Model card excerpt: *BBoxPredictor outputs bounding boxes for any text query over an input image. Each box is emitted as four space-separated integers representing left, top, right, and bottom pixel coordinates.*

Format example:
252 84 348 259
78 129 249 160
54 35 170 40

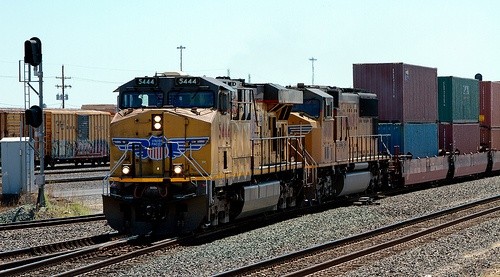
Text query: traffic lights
25 105 43 129
24 37 44 69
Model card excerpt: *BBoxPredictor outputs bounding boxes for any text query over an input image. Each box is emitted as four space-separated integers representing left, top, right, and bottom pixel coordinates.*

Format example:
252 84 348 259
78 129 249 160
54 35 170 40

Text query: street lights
309 58 318 85
177 46 188 72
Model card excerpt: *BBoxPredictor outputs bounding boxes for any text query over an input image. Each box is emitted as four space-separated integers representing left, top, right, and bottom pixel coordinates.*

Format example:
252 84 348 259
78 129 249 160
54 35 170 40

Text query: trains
100 62 500 242
0 106 112 167
